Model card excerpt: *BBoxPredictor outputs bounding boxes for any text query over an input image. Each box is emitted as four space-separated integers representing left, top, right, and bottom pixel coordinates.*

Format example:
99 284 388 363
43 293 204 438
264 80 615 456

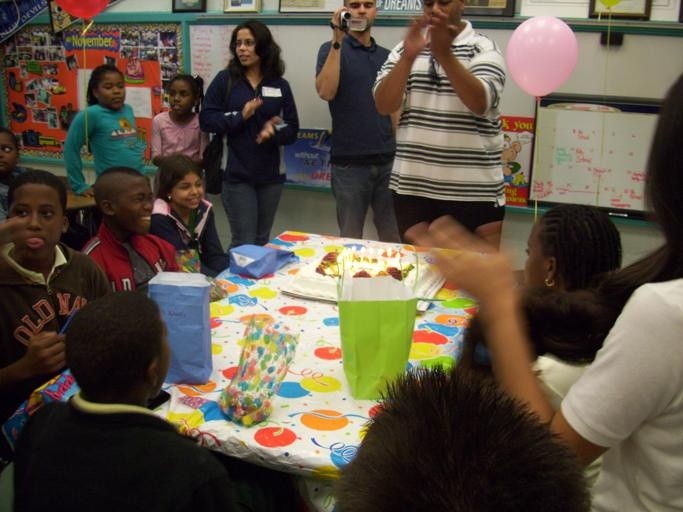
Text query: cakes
286 244 437 308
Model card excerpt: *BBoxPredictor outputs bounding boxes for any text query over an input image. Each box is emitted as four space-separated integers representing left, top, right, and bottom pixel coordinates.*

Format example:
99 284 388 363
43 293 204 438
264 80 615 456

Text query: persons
62 63 159 197
333 362 592 512
199 19 300 251
11 289 304 512
314 0 403 244
2 171 113 418
1 214 44 244
403 72 682 512
372 1 506 252
82 167 228 300
150 155 230 277
520 204 622 413
149 73 211 167
0 126 32 219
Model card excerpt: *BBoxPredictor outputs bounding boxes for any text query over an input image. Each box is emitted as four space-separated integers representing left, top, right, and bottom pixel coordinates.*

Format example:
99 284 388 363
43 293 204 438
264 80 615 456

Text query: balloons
505 15 579 99
54 0 110 20
598 0 621 10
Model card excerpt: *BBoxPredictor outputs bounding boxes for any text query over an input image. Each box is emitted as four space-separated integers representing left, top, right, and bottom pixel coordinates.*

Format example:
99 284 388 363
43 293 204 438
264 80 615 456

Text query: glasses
428 55 440 81
236 41 255 47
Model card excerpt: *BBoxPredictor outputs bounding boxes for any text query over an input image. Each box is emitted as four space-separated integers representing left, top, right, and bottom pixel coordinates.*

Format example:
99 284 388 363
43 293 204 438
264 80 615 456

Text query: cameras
340 10 368 32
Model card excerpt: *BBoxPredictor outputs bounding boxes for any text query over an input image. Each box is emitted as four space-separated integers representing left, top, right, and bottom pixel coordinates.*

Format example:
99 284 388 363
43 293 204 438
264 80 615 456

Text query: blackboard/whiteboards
181 15 683 230
528 93 665 219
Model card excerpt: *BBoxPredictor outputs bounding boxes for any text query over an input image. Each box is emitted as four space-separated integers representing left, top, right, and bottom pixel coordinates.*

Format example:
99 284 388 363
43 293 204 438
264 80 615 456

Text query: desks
65 192 99 211
2 231 479 512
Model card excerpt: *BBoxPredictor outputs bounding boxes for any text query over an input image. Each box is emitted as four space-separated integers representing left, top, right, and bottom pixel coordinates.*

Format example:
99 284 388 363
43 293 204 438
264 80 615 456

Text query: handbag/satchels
202 135 222 195
336 243 420 400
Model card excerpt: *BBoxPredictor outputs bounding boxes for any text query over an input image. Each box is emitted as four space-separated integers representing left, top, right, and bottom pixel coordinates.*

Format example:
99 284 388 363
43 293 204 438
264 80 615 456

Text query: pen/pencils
60 309 77 334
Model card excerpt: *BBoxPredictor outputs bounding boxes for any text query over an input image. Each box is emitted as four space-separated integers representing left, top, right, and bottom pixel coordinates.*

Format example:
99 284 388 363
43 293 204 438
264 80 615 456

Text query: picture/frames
589 0 652 20
279 0 345 13
172 0 206 12
224 0 261 13
461 0 514 17
